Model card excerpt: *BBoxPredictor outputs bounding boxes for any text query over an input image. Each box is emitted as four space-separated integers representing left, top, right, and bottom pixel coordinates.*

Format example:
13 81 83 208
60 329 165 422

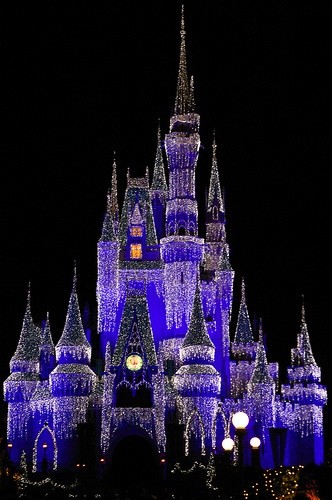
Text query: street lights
219 436 235 500
231 410 249 500
249 436 261 466
